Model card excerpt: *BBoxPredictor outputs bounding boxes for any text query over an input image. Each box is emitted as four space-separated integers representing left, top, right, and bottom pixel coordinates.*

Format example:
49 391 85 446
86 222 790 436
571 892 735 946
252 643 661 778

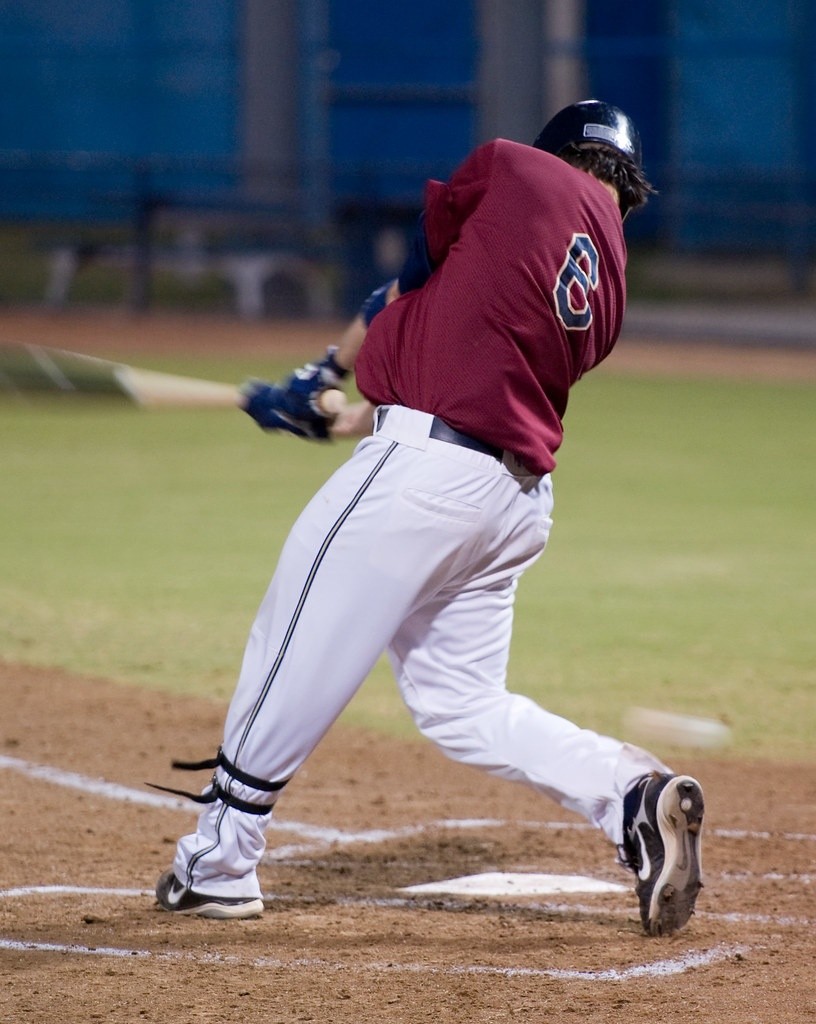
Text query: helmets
532 99 643 165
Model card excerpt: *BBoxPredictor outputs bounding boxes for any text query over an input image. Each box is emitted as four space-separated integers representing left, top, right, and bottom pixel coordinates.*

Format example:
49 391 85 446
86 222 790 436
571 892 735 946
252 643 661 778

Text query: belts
377 407 504 460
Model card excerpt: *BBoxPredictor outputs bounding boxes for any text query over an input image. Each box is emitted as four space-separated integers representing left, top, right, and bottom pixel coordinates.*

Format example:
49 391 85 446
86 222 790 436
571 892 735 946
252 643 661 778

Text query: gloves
285 344 350 394
233 376 337 443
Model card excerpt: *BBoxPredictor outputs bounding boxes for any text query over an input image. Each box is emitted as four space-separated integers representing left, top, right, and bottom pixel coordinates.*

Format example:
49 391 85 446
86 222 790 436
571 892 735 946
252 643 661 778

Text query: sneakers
155 866 265 920
616 770 704 940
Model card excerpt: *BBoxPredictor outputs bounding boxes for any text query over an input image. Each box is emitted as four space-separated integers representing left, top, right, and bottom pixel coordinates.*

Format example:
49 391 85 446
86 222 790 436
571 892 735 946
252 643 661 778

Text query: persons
155 100 704 938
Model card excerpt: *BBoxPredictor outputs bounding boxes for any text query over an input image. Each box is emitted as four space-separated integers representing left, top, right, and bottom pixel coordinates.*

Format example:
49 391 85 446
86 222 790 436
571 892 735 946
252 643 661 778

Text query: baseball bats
1 341 350 416
